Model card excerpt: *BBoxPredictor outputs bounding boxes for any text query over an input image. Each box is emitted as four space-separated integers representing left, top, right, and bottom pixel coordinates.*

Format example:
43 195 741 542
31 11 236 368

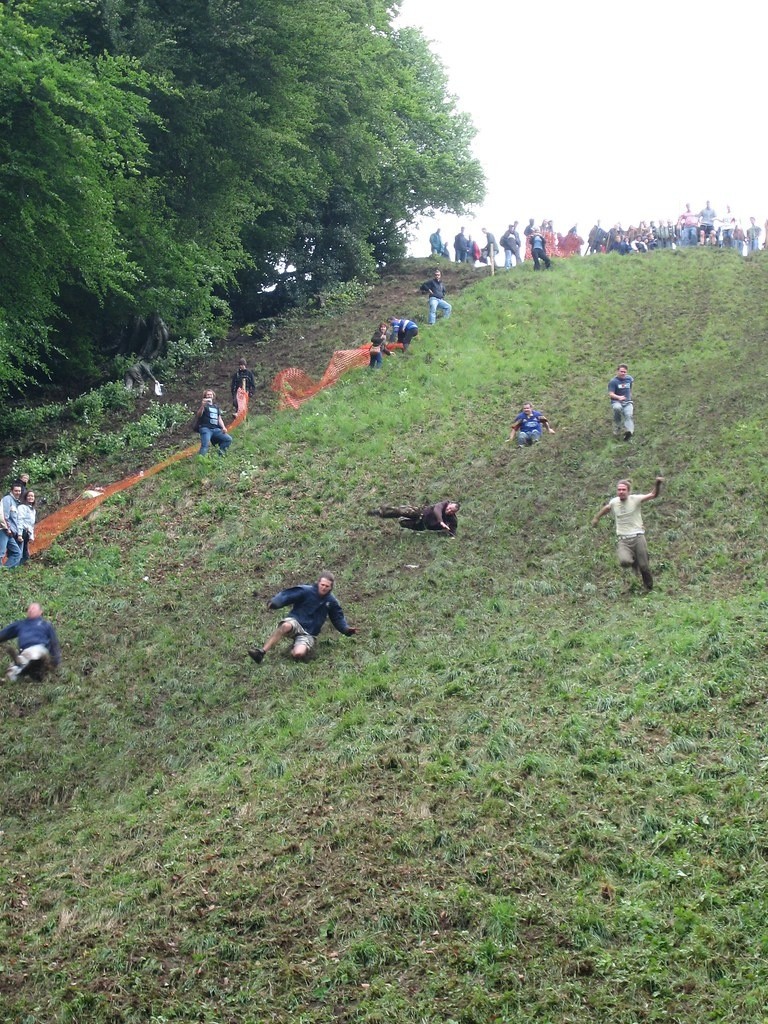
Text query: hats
238 358 247 365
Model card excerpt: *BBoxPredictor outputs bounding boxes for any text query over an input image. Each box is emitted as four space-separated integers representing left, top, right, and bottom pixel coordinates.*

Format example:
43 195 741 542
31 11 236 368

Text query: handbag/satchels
370 346 380 356
155 382 163 396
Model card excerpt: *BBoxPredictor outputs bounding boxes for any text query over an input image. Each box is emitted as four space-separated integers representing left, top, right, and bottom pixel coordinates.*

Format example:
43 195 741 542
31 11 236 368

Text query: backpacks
192 404 218 433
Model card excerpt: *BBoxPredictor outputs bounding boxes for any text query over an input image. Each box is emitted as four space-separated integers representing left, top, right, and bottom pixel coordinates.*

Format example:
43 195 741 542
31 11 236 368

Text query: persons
367 501 460 539
0 601 61 682
505 401 555 448
231 358 255 418
592 477 665 591
422 270 452 325
0 473 36 568
368 316 418 368
248 572 361 665
430 200 761 271
608 364 634 440
197 388 232 456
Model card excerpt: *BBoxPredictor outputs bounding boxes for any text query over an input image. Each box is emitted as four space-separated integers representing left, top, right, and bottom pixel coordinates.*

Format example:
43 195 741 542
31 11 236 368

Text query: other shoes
7 647 22 667
248 649 263 664
139 393 145 399
624 431 631 441
367 508 381 516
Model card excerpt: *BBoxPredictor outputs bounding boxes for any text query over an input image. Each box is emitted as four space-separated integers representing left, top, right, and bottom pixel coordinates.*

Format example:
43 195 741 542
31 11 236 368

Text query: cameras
205 399 212 403
